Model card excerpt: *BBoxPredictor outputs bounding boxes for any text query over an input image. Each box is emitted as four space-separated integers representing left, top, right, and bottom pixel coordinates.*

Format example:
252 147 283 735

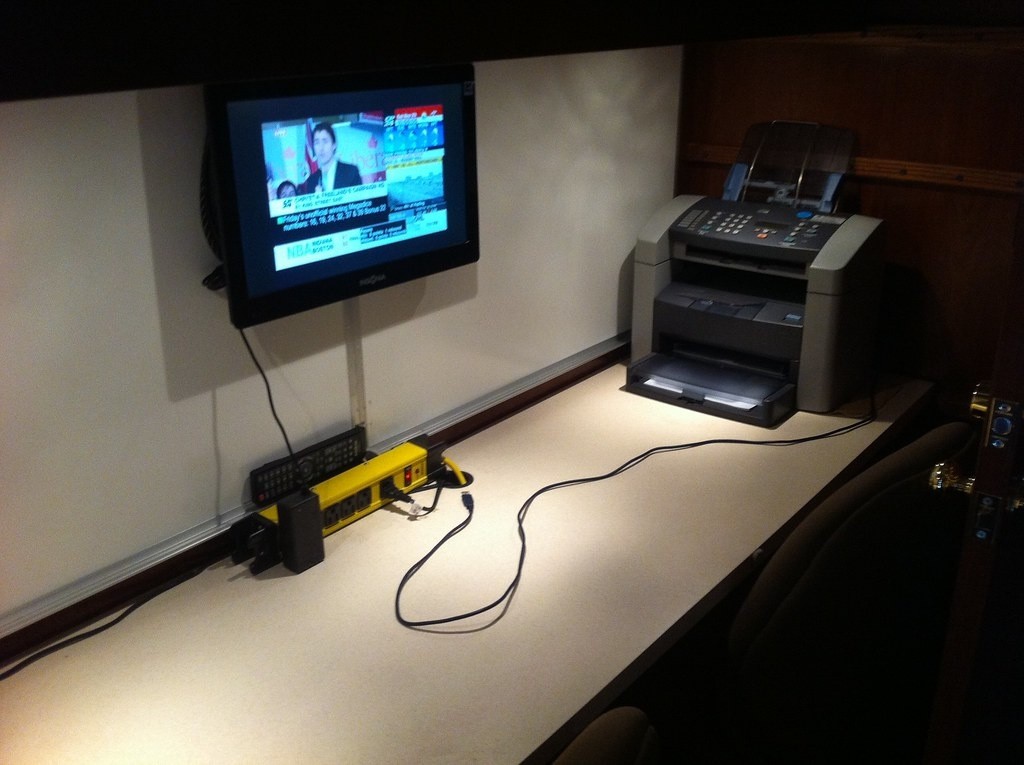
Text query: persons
300 122 361 195
277 182 297 200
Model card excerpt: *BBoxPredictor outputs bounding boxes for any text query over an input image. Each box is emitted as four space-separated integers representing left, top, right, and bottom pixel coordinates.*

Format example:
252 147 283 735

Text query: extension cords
253 434 447 551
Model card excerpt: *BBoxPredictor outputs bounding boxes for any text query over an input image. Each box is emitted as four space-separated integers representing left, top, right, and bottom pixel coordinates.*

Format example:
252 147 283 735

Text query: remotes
248 424 368 509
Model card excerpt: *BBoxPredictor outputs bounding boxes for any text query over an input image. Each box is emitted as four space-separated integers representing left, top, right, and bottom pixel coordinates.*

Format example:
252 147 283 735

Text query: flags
303 118 318 181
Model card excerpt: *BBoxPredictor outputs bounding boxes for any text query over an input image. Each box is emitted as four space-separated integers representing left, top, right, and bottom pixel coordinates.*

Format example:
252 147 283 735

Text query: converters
271 489 328 575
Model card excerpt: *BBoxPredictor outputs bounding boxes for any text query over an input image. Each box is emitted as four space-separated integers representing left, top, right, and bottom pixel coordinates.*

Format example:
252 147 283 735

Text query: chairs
552 706 659 765
726 421 978 663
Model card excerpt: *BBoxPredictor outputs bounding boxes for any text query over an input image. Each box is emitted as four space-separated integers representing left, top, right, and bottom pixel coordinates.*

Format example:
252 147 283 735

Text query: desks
0 352 932 765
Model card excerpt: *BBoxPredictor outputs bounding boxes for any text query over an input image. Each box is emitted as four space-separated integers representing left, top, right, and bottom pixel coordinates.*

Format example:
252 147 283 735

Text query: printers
619 116 888 430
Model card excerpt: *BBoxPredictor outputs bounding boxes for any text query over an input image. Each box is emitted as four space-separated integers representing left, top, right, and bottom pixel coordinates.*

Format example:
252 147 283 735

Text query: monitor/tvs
203 61 482 332
